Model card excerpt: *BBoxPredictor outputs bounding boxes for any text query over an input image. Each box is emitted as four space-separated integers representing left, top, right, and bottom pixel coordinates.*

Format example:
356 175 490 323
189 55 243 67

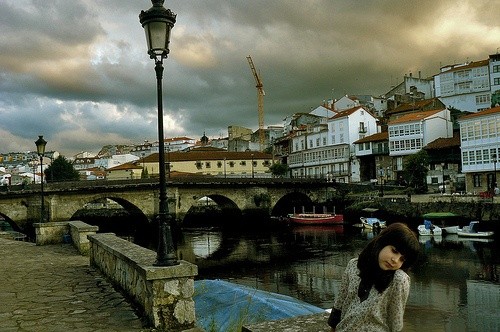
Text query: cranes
244 54 267 153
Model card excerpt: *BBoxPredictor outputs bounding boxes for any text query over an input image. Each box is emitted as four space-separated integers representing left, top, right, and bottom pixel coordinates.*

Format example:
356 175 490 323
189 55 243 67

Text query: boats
417 219 460 236
286 213 344 225
359 216 387 230
456 220 495 238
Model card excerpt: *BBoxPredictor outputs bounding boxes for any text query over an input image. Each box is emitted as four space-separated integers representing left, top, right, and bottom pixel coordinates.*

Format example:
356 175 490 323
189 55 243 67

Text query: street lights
34 135 48 223
138 0 182 267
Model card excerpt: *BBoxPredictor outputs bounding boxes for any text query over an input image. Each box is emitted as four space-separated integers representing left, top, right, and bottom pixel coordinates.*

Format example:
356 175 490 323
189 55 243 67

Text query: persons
327 222 421 332
